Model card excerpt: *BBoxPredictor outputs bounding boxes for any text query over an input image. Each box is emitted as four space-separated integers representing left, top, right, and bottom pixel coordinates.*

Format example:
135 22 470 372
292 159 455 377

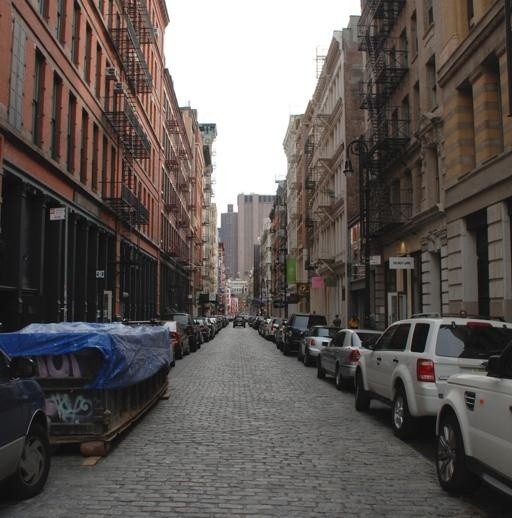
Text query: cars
247 315 288 350
128 312 232 359
300 325 340 368
233 316 246 329
318 329 386 390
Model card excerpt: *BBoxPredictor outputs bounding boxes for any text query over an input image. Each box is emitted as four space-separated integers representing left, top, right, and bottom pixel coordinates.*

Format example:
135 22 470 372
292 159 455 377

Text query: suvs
281 314 329 356
352 312 509 438
0 349 57 506
434 344 512 496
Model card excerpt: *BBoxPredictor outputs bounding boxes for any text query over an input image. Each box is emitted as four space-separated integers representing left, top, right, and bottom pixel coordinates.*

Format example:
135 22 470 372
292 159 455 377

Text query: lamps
342 140 368 178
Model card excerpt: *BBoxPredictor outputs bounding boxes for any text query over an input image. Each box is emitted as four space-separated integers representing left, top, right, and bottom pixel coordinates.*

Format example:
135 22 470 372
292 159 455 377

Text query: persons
333 314 342 328
348 315 359 329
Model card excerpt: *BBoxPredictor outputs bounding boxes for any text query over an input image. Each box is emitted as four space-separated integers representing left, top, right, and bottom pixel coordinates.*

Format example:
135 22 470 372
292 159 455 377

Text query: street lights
279 244 289 316
342 138 374 328
